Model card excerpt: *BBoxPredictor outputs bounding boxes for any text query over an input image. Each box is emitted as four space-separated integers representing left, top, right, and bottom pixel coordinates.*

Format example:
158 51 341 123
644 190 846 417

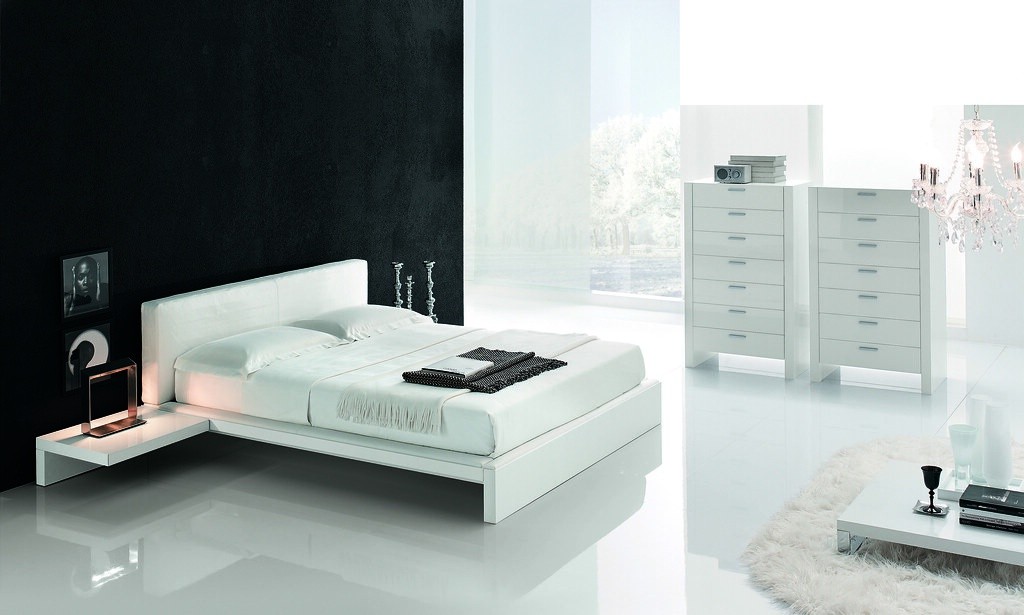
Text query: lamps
909 104 1024 258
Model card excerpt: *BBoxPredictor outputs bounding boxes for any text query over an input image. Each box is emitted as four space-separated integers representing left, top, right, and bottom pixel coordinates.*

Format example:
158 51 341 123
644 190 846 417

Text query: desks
837 456 1024 567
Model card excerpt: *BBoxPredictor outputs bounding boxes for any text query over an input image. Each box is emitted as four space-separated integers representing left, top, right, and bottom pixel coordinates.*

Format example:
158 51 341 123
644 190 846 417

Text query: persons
70 257 109 307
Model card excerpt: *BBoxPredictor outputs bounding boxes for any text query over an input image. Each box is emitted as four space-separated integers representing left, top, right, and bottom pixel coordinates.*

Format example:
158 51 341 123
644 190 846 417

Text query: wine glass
921 466 943 513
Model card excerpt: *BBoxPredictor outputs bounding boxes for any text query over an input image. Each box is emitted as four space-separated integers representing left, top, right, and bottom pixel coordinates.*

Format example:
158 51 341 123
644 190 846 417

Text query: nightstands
36 404 209 487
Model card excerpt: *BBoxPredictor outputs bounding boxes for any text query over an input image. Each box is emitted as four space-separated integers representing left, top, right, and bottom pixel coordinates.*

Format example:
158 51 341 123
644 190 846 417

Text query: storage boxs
938 469 1024 502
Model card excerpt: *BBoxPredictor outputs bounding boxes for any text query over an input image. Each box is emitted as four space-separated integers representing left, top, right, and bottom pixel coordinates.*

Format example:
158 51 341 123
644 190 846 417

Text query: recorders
714 165 751 183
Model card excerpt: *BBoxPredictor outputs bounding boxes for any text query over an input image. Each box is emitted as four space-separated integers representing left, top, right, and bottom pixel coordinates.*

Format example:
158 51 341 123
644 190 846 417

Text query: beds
138 259 663 524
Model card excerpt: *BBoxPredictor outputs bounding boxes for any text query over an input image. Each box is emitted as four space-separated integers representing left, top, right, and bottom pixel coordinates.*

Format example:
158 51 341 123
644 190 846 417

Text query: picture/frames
63 323 113 393
58 246 113 323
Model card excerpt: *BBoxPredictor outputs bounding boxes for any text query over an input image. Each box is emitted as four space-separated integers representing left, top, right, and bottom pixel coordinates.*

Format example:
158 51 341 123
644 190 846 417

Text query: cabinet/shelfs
809 185 948 396
684 176 810 384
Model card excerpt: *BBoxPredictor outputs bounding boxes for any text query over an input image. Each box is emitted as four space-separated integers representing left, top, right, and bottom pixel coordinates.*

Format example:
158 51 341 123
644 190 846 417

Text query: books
727 154 787 183
957 484 1024 534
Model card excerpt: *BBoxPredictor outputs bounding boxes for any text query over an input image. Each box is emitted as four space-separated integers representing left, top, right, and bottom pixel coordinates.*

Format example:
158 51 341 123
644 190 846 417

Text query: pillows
291 302 433 344
172 323 349 380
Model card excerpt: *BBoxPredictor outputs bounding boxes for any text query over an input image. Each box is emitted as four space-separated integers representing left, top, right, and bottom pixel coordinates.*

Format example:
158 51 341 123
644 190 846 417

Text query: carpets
744 433 1024 615
